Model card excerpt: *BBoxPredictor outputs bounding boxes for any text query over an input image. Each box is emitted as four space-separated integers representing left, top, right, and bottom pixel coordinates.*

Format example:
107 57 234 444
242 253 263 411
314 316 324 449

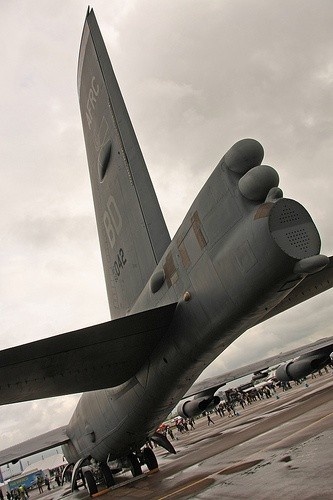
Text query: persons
207 412 215 426
256 386 271 400
294 377 306 386
162 430 166 436
45 474 51 490
6 484 30 500
175 418 195 433
146 441 157 449
53 467 71 486
318 370 322 376
167 427 175 440
324 367 328 373
215 404 236 417
271 382 278 399
37 478 43 494
311 373 316 379
328 364 333 369
239 394 257 409
281 382 292 392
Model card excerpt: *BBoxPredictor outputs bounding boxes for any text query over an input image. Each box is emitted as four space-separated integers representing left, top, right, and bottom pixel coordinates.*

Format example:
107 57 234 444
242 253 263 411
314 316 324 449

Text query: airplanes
0 3 333 496
